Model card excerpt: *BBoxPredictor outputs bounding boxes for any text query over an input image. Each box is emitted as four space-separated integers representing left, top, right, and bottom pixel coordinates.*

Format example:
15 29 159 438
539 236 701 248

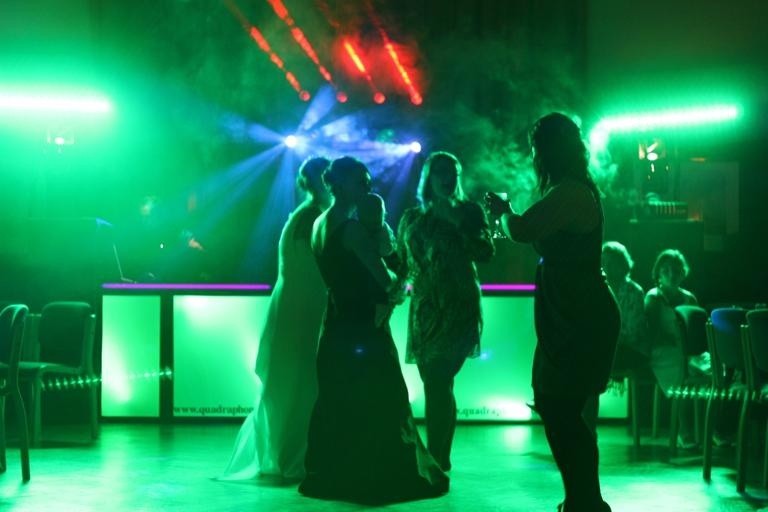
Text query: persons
235 158 335 481
355 193 403 329
638 247 699 310
480 111 624 512
396 149 496 472
301 154 452 505
523 237 645 416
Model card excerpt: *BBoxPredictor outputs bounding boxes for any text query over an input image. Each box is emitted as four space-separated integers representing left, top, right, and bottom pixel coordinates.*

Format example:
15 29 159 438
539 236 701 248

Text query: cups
488 191 508 240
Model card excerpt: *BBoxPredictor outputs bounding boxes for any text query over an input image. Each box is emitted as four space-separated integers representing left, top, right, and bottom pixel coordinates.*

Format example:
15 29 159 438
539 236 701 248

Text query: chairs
622 304 767 494
0 301 98 483
